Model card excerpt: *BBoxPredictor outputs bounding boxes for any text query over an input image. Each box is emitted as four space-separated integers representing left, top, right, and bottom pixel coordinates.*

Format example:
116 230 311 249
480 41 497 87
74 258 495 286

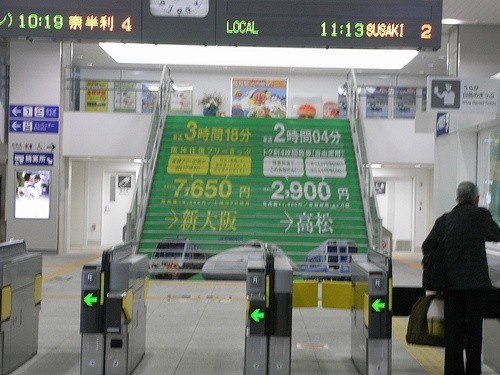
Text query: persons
23 173 39 199
33 175 43 197
420 181 499 375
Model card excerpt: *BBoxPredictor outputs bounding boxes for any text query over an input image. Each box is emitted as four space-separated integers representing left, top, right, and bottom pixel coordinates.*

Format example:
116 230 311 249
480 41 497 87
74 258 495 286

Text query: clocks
149 0 209 18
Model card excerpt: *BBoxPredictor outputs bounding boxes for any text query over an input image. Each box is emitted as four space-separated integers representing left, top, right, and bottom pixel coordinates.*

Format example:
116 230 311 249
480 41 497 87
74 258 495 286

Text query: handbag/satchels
405 252 447 347
